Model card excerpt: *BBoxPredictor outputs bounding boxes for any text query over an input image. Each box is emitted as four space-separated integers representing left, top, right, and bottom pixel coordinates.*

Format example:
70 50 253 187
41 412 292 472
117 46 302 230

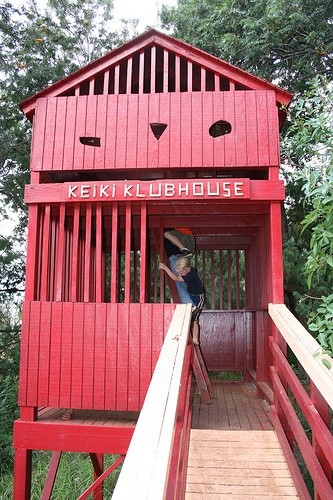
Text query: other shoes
180 249 193 257
192 307 202 320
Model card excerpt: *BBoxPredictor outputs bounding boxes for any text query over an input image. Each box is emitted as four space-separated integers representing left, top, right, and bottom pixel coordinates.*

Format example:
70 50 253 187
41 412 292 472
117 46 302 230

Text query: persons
159 257 205 345
163 227 200 316
